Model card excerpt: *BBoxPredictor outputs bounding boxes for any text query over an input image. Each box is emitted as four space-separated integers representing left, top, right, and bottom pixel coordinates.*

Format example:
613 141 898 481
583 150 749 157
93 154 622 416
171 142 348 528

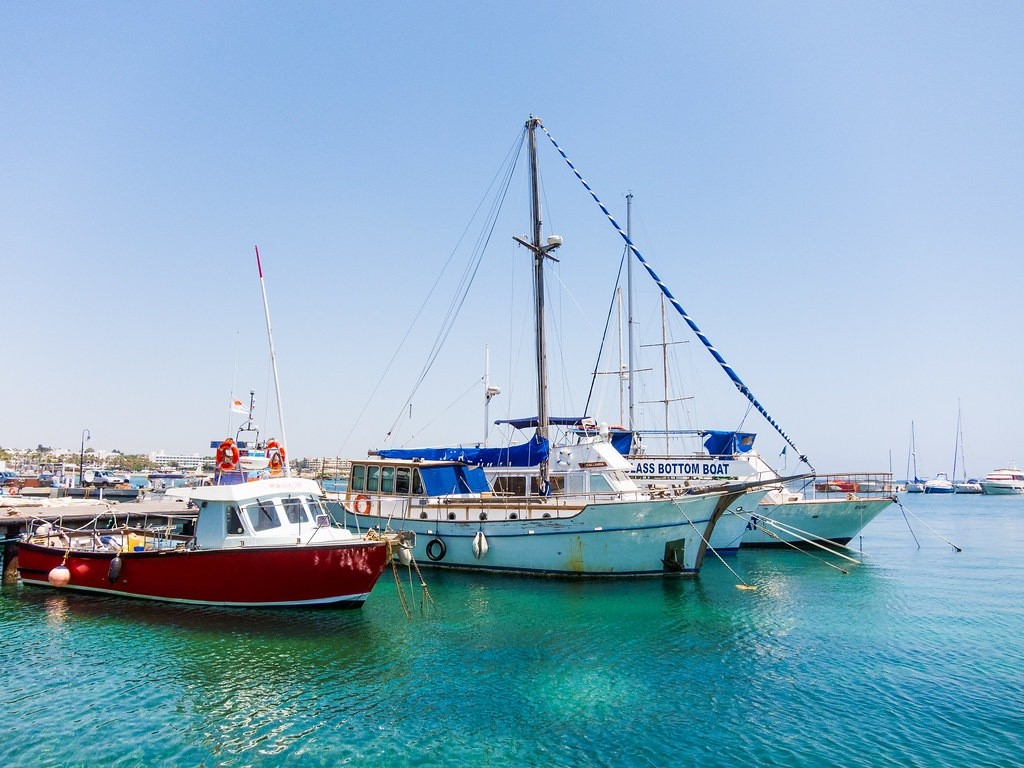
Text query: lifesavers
557 449 572 466
216 441 239 471
265 441 286 468
9 487 16 495
353 495 370 514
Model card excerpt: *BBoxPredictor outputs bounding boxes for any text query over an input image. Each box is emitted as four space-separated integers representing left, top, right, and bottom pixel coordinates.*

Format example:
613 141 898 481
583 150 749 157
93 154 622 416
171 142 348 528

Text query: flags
230 391 251 414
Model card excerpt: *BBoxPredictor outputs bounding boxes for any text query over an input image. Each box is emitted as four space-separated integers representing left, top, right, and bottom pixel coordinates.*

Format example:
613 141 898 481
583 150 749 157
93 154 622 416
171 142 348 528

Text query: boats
979 459 1024 495
950 398 984 493
562 287 896 551
811 419 925 493
1 114 816 607
924 473 956 493
